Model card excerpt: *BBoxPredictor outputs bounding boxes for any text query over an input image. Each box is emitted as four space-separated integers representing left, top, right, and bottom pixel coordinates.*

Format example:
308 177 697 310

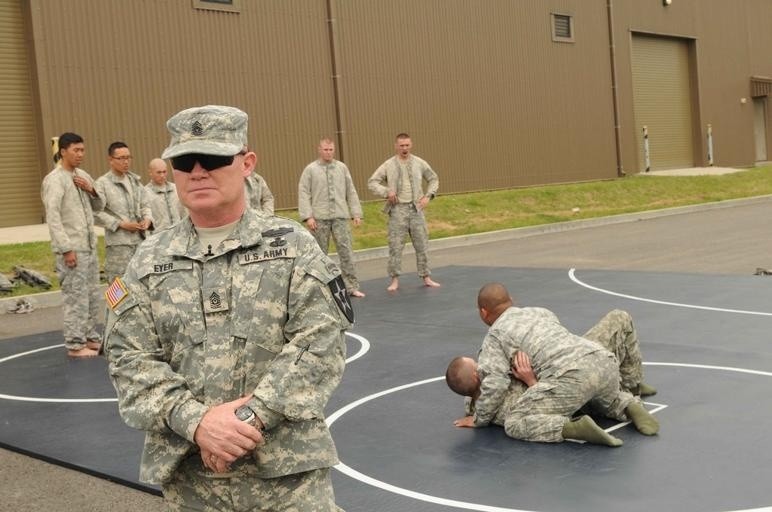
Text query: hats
161 103 251 160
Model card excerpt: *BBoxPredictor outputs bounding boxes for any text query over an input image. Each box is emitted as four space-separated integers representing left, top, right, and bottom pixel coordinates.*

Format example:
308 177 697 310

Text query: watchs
235 405 263 435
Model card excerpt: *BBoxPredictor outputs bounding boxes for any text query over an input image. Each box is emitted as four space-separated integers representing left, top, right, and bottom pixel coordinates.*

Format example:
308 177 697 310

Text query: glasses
170 154 235 173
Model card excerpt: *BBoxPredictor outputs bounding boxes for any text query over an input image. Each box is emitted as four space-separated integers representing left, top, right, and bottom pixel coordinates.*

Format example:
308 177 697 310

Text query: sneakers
5 294 37 314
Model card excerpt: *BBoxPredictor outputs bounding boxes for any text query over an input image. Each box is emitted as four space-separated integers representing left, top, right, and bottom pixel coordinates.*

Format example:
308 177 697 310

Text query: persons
40 132 188 357
446 308 657 426
367 133 441 292
104 105 356 512
297 137 364 298
246 173 275 217
453 283 659 448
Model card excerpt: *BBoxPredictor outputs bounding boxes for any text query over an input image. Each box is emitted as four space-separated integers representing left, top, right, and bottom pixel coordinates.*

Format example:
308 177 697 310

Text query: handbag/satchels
0 271 21 290
12 262 55 292
